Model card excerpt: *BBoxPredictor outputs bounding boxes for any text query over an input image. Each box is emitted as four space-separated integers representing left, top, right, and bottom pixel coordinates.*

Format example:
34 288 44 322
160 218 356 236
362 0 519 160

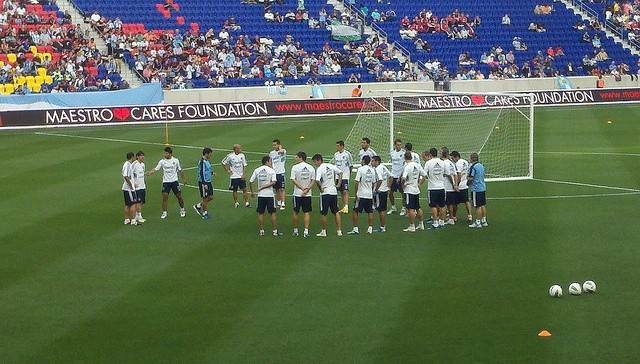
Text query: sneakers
316 229 327 237
192 204 203 215
468 215 473 221
337 230 343 237
347 227 359 235
416 224 424 230
426 216 433 221
124 218 130 224
161 211 168 219
469 222 482 228
234 202 240 208
426 220 434 224
272 229 283 236
482 221 488 226
427 224 441 230
373 226 386 233
202 212 211 220
180 210 186 217
403 226 415 232
386 207 397 215
399 207 406 216
277 201 281 208
439 224 444 228
280 202 286 211
131 219 142 226
138 217 146 223
259 230 265 236
246 202 253 208
366 226 373 234
339 207 345 213
304 229 309 237
293 228 299 236
343 207 348 214
444 219 455 225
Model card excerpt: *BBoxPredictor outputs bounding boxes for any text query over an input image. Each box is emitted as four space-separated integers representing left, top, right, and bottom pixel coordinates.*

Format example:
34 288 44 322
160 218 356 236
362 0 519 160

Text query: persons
269 138 287 210
191 147 217 220
346 154 378 234
289 151 316 237
1 0 126 94
466 152 489 228
597 74 605 88
404 141 420 163
349 0 589 81
311 153 344 236
221 143 250 208
249 155 284 236
145 146 188 218
399 151 428 232
334 139 354 213
120 151 138 225
351 84 362 96
358 136 377 162
590 0 640 74
437 146 459 225
369 155 394 232
419 147 451 228
386 138 412 215
126 0 349 87
131 149 147 223
449 150 473 222
421 150 431 161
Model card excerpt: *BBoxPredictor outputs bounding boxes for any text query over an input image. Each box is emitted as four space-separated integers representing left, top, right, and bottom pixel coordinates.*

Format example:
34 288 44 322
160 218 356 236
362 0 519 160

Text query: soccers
583 280 597 293
568 282 582 295
550 285 563 296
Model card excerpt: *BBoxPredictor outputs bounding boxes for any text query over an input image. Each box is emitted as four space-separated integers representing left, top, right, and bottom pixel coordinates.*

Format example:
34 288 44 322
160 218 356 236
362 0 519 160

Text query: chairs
0 0 130 94
574 0 612 26
